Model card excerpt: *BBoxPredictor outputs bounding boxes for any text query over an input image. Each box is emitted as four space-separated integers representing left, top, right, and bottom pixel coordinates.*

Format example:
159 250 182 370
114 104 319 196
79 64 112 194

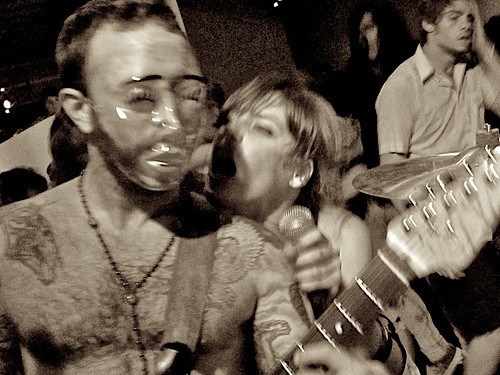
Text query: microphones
280 203 330 328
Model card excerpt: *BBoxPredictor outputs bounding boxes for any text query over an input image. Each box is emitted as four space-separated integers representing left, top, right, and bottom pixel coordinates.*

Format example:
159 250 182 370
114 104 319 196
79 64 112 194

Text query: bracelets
483 43 496 74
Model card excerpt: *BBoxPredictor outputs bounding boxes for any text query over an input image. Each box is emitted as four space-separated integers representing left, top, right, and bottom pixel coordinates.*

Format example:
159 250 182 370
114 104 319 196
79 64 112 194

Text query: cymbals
352 146 488 202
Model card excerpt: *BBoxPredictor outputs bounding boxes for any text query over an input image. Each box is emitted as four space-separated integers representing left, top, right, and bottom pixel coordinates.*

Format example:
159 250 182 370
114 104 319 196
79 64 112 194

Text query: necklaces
79 166 186 375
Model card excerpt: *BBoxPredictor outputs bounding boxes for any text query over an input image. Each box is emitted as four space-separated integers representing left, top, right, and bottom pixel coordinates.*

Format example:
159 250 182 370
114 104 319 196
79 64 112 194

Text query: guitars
264 148 500 375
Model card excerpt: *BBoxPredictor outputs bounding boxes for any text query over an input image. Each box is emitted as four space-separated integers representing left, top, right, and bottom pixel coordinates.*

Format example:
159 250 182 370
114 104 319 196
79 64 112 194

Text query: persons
0 87 90 210
205 69 420 375
374 0 500 338
0 0 388 375
359 8 413 62
